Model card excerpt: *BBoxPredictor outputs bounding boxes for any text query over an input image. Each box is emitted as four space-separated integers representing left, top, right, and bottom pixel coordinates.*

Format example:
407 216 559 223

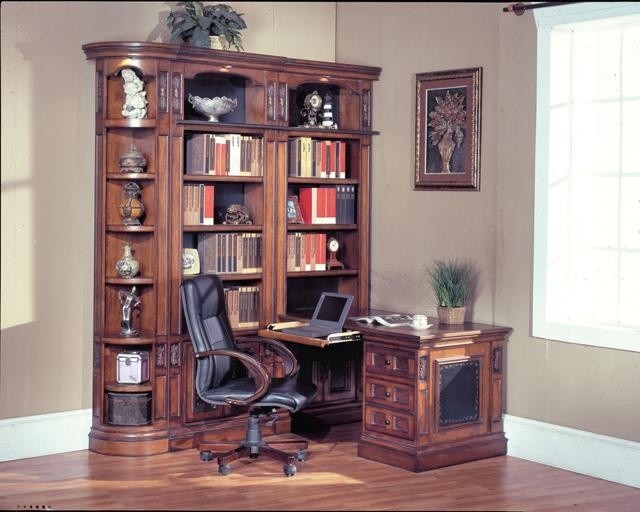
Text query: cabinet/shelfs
82 41 170 458
171 46 277 453
276 58 382 435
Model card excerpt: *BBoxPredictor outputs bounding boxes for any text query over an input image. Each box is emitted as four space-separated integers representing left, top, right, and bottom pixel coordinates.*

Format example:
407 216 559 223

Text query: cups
413 315 428 325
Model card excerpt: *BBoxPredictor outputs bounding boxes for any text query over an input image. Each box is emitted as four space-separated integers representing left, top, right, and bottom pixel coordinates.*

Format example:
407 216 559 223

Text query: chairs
178 275 307 477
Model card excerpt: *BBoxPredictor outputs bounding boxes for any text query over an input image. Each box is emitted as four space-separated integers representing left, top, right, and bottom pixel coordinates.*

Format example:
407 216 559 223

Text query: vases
438 133 456 173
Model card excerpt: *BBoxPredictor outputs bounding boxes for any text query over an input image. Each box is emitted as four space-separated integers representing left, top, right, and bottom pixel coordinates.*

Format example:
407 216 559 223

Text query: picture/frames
411 66 483 191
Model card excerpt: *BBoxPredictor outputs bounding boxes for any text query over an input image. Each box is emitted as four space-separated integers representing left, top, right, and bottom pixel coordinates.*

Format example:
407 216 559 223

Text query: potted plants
164 1 248 52
423 255 478 324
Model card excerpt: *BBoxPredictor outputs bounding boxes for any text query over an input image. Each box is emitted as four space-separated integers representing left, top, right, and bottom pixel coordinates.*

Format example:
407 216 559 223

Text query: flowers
427 90 466 148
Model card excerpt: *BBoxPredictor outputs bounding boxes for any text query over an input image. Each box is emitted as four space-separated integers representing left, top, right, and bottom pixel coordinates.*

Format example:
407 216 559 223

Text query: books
350 311 411 327
187 132 263 330
288 136 355 272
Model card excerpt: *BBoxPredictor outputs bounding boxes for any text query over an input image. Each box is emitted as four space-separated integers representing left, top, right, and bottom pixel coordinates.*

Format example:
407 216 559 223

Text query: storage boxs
116 351 149 385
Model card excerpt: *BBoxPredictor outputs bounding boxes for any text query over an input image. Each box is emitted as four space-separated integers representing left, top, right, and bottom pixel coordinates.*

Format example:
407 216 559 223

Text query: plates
408 324 433 330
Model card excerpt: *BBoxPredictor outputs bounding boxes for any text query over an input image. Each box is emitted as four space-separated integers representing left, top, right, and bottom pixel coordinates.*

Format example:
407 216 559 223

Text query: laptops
282 291 354 338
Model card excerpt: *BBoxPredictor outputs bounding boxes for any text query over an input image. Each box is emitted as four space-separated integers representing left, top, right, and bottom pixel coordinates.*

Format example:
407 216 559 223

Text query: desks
277 307 513 473
